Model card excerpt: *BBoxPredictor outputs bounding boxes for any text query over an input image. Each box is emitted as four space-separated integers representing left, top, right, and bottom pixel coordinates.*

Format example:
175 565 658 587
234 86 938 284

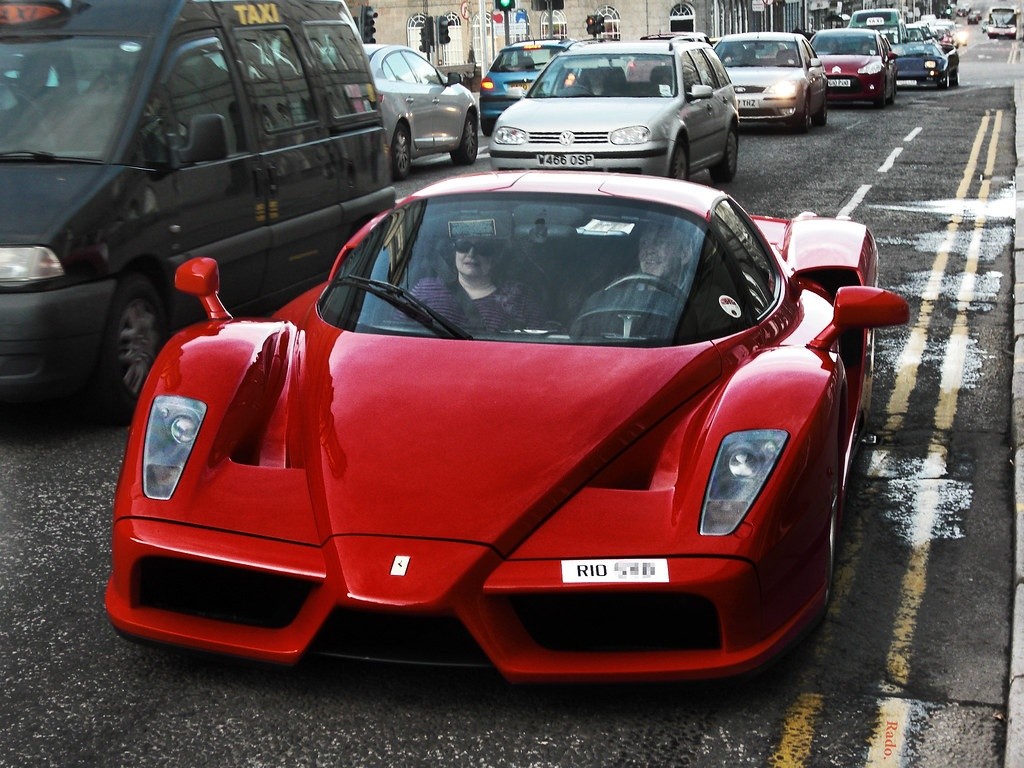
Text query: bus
986 7 1020 41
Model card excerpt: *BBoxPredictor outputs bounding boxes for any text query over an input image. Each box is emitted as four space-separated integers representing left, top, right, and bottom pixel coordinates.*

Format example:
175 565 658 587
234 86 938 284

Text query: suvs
479 36 582 139
487 34 740 186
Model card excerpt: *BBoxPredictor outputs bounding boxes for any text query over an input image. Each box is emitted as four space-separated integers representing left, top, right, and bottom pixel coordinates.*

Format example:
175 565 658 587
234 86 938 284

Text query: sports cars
102 166 914 692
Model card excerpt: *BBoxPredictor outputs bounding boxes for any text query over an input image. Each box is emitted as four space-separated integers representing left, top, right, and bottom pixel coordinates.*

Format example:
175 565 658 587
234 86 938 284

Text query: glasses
452 239 498 253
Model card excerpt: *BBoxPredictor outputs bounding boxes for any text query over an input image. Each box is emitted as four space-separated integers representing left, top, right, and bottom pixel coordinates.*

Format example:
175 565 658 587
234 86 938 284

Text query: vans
0 1 401 424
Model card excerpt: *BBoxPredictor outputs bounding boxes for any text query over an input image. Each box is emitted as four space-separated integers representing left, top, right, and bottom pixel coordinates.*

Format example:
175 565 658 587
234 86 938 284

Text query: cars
809 27 900 107
706 32 831 135
966 14 978 26
973 10 982 19
982 21 989 33
626 32 715 84
353 45 482 178
844 4 972 90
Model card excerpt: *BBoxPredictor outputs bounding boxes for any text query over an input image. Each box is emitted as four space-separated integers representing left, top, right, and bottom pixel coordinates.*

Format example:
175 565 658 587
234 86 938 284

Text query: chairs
597 67 626 95
519 56 534 70
646 66 672 94
433 210 546 322
740 47 756 64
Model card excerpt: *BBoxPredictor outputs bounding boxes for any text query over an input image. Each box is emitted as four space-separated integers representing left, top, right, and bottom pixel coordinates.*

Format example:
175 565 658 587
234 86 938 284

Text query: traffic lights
417 27 424 53
423 16 434 47
594 16 606 35
495 0 515 11
586 16 596 37
438 16 450 45
358 4 377 46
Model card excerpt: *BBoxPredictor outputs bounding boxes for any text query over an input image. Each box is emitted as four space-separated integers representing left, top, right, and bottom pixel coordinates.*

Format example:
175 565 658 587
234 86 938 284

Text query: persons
579 62 619 96
604 219 729 339
397 216 548 334
